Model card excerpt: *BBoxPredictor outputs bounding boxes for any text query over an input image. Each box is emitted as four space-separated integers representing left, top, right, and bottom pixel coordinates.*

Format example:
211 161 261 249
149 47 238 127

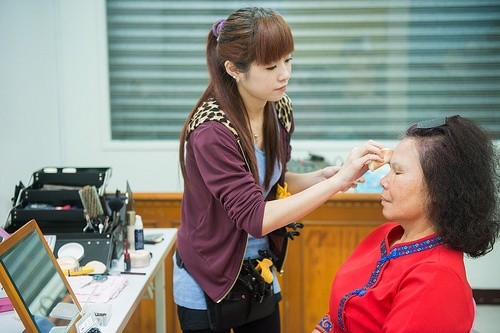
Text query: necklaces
251 127 263 142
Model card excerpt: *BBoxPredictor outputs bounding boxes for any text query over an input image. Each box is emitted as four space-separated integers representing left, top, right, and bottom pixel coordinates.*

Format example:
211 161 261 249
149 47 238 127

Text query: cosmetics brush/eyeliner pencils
83 200 123 233
120 272 146 275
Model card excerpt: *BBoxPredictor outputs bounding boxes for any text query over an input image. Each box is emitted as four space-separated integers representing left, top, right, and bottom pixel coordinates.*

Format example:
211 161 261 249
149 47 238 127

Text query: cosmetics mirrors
0 219 84 333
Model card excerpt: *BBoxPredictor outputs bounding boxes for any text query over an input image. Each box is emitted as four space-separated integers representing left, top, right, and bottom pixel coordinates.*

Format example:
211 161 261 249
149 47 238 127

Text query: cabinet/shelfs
123 193 394 333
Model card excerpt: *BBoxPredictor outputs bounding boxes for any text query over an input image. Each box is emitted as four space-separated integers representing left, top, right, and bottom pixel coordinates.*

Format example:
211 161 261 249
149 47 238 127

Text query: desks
0 228 179 333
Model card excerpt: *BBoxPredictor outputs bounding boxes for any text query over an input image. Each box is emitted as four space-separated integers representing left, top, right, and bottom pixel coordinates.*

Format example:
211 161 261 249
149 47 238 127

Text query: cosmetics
367 147 395 172
124 211 152 272
55 256 95 277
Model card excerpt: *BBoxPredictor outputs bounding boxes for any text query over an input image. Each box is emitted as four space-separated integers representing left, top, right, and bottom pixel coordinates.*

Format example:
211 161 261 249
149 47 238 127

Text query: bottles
127 211 137 250
133 215 144 250
123 250 131 273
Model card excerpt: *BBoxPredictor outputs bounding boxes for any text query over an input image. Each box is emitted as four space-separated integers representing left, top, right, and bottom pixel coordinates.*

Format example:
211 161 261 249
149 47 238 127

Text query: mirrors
0 219 82 333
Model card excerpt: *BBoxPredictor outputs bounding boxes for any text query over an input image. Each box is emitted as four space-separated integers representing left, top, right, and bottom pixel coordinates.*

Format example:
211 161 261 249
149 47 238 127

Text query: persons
309 115 500 333
171 7 388 333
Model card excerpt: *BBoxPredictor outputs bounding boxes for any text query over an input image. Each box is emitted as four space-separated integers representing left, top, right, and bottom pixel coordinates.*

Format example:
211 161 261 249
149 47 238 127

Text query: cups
129 250 151 268
